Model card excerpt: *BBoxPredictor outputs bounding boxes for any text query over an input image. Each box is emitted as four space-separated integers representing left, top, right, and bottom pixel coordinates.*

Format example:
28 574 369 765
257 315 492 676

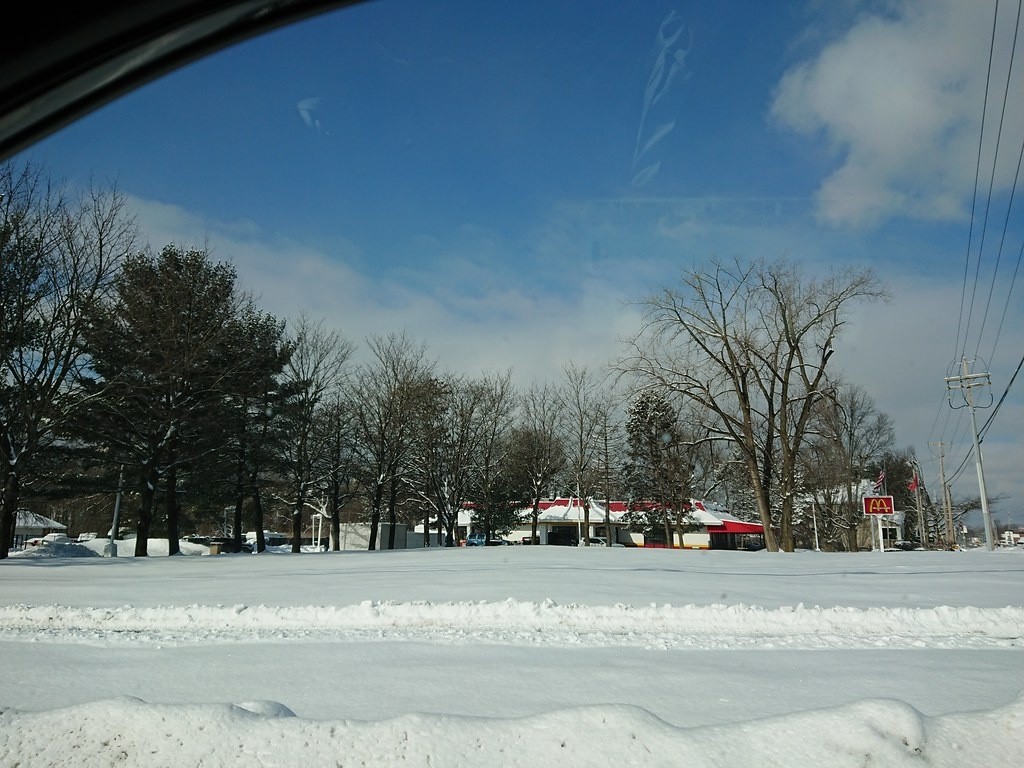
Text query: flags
874 469 885 490
908 473 918 491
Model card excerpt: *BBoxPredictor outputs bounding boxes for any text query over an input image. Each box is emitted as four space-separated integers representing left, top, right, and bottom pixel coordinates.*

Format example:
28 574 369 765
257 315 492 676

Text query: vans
466 532 508 546
108 526 133 540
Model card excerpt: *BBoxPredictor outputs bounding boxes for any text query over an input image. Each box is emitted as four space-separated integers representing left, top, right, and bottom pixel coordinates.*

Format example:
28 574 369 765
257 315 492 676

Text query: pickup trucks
522 532 565 544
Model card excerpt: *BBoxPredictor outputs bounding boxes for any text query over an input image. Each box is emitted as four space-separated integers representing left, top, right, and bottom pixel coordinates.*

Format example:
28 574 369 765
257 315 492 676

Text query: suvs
577 537 625 547
